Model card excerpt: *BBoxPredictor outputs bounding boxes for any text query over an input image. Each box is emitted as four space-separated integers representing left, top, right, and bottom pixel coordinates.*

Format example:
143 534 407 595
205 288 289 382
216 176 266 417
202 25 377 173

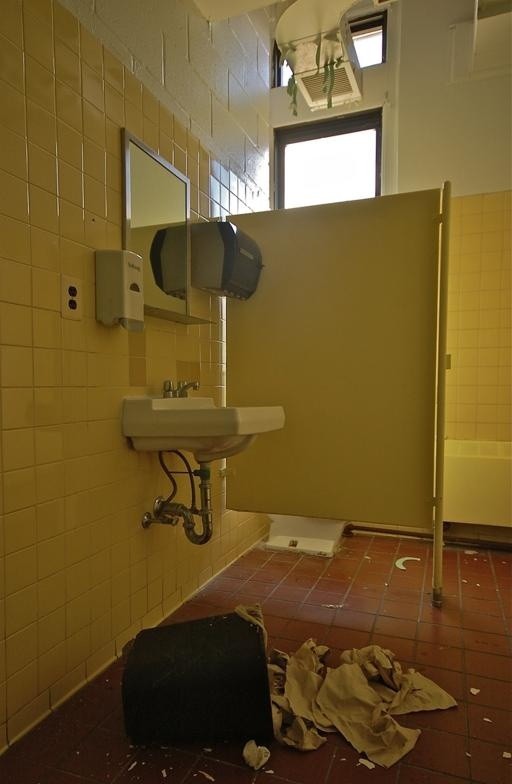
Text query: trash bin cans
122 600 277 751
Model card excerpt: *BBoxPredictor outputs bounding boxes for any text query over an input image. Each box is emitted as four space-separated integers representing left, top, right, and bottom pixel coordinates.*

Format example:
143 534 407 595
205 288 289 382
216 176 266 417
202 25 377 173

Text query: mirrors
119 127 189 326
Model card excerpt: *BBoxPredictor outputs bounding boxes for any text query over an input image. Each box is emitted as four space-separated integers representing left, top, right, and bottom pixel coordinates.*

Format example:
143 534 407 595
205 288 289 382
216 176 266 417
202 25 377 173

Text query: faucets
162 377 206 401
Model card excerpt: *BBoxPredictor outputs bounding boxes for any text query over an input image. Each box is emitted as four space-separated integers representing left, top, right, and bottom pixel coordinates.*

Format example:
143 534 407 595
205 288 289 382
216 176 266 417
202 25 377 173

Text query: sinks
121 395 286 464
